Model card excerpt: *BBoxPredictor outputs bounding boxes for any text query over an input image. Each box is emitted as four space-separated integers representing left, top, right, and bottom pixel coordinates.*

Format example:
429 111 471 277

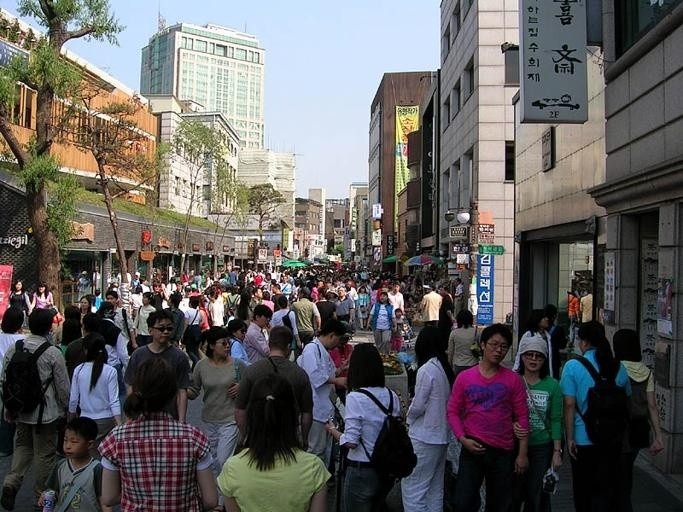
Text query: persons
0 259 664 512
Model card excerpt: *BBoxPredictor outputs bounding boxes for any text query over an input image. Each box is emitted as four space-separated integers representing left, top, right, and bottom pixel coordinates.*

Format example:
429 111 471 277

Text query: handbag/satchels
469 340 482 357
360 304 365 313
282 315 294 330
635 419 652 449
550 433 565 459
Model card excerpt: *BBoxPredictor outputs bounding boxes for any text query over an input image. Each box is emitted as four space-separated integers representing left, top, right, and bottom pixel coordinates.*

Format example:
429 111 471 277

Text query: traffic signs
478 244 506 255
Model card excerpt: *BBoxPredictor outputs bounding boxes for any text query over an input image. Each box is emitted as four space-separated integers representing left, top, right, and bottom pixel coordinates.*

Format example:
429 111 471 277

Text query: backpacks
572 354 630 445
3 339 54 415
354 387 417 477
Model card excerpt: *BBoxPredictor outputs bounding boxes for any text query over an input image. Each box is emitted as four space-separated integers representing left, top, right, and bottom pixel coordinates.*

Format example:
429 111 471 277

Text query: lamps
444 204 477 224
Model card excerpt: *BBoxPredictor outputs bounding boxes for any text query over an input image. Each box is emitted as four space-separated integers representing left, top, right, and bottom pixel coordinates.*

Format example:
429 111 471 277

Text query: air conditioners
372 203 382 220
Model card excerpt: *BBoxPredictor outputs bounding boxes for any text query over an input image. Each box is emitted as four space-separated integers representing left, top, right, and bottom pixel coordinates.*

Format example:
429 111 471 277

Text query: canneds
43 489 55 512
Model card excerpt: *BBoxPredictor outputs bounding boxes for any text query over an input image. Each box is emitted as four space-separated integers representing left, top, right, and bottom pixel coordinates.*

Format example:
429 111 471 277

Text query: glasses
150 325 174 332
238 328 247 334
486 341 509 352
215 340 233 346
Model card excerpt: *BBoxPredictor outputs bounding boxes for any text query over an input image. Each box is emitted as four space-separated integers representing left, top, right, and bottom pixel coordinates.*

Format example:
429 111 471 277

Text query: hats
519 336 548 360
